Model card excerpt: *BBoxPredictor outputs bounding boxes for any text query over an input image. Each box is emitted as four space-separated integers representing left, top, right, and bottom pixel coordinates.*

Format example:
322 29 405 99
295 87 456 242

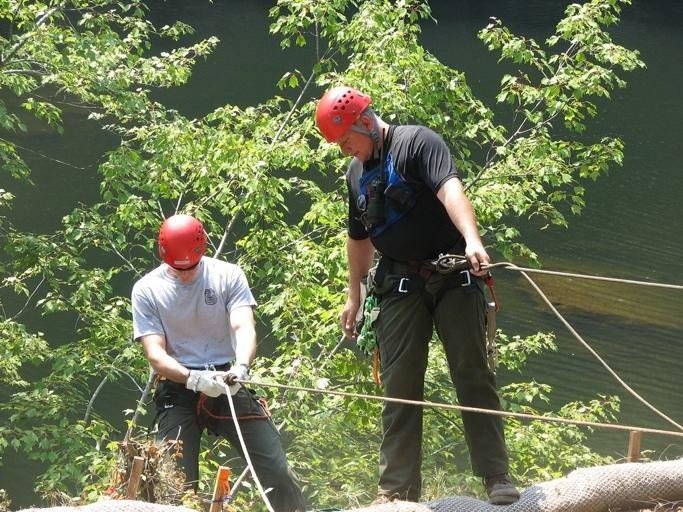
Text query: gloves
186 364 249 399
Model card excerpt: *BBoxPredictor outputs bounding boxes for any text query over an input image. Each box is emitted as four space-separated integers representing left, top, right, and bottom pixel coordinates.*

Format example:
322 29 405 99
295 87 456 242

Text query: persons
129 213 308 512
316 85 522 506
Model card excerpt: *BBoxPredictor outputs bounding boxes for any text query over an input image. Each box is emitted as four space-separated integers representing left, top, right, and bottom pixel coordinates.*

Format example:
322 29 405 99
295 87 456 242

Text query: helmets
158 214 208 270
316 86 372 144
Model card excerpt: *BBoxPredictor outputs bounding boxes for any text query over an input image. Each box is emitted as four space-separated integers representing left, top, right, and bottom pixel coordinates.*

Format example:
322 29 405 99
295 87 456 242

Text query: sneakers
370 495 419 506
483 473 520 503
